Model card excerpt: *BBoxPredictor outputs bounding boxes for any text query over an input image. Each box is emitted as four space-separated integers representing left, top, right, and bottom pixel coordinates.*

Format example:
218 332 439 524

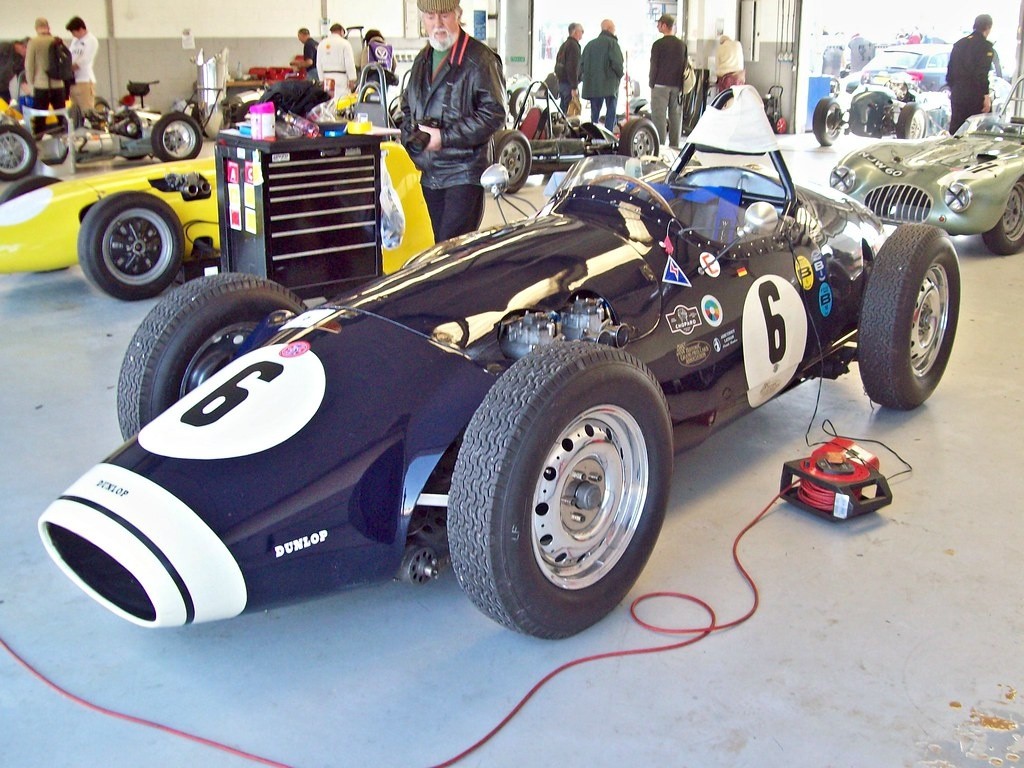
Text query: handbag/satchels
683 66 696 94
567 93 582 115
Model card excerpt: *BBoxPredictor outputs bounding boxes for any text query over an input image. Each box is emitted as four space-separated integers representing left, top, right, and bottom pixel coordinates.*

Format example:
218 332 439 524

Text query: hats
417 0 460 14
656 15 674 26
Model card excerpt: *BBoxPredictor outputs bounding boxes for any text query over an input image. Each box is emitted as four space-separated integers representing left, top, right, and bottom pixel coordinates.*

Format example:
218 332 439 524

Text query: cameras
404 117 445 157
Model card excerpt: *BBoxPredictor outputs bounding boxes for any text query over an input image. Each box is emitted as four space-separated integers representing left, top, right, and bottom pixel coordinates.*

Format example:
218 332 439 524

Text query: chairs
664 84 796 219
354 62 390 141
513 80 553 140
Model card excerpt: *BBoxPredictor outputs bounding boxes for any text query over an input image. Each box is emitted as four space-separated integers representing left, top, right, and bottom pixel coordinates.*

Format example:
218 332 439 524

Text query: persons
946 14 994 135
291 28 319 84
360 31 397 92
649 15 688 147
715 38 745 107
576 20 624 132
0 38 31 105
400 0 507 243
25 18 70 134
315 24 356 97
65 16 99 116
554 23 584 114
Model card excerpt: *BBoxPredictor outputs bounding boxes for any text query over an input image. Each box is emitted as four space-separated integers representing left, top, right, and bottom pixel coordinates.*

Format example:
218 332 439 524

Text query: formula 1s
0 64 962 638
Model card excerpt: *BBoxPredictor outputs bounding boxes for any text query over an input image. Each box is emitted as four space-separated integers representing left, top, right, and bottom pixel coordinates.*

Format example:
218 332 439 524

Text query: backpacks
44 36 73 80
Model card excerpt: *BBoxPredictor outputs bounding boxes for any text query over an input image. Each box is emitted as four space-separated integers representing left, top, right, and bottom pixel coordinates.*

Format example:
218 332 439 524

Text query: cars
812 43 1011 145
829 78 1024 253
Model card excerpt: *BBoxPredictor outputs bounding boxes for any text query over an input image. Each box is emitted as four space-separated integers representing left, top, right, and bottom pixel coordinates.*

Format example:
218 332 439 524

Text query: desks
227 80 275 92
